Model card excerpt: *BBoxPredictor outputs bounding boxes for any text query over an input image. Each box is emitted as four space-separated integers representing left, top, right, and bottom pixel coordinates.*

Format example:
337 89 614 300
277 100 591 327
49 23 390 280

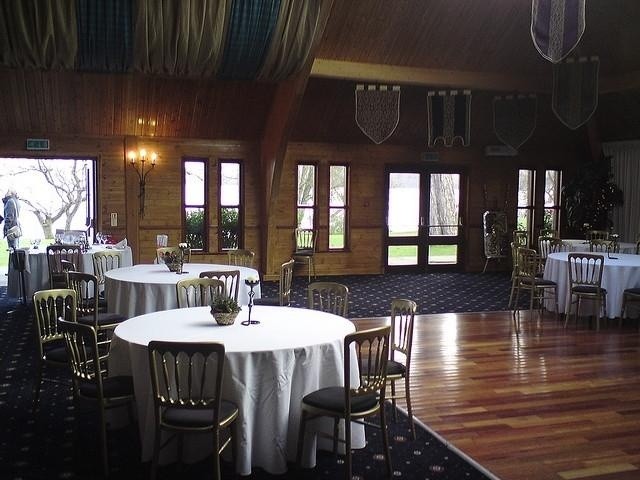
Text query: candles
247 274 258 283
140 149 146 160
128 151 136 163
151 154 156 164
583 222 590 227
178 242 188 249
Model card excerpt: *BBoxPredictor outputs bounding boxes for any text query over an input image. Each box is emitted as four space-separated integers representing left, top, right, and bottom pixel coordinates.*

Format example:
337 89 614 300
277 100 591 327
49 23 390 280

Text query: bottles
58 240 62 246
79 236 84 251
82 237 86 250
85 241 89 249
54 240 58 247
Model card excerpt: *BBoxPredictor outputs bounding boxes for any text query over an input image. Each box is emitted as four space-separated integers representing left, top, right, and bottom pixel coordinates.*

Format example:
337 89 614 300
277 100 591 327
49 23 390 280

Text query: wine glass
36 239 41 248
101 234 107 249
31 239 36 249
96 232 102 248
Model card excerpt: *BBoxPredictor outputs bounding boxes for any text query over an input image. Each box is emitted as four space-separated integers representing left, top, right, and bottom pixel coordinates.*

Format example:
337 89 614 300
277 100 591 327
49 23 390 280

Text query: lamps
241 273 260 326
176 242 189 274
582 222 592 244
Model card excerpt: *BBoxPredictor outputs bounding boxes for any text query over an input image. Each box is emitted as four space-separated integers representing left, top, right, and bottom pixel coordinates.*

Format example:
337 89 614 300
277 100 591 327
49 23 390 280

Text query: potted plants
164 256 184 272
209 294 243 325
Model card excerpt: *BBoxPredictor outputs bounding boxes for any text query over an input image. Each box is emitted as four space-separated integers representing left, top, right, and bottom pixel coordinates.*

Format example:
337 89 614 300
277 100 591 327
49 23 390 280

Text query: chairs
355 298 417 438
147 341 239 480
297 324 393 480
292 228 318 283
59 316 136 480
199 270 241 308
177 278 226 310
227 249 255 268
156 247 191 264
508 229 639 329
46 245 82 289
253 260 292 306
481 223 509 275
308 282 349 317
92 252 129 299
67 270 129 355
32 288 78 403
